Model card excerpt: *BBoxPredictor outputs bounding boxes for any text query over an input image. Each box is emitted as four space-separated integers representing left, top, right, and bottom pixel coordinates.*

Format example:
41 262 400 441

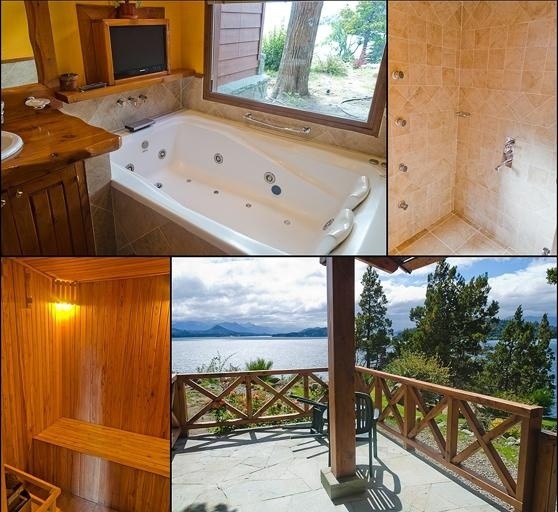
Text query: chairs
283 392 380 468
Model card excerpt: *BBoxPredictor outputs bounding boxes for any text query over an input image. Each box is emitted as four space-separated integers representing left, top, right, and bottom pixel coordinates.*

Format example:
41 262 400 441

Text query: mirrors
1 0 61 97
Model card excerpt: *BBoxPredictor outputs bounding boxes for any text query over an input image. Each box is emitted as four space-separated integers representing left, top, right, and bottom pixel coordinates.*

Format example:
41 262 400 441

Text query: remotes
79 81 105 90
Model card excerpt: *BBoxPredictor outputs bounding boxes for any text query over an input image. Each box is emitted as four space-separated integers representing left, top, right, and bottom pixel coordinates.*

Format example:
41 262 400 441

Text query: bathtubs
108 106 387 255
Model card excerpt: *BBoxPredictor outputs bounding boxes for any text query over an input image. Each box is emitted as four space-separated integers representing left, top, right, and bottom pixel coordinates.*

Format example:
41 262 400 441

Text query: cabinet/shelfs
0 161 95 256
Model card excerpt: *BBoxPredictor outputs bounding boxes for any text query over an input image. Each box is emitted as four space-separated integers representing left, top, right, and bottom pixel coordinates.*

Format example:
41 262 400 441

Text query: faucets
495 159 510 172
0 99 5 124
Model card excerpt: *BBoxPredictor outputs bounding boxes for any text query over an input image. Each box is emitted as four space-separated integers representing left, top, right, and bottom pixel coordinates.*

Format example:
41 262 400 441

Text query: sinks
0 130 25 160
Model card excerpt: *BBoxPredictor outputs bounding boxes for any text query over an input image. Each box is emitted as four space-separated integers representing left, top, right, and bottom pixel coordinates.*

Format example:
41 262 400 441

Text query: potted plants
60 72 80 91
114 0 142 19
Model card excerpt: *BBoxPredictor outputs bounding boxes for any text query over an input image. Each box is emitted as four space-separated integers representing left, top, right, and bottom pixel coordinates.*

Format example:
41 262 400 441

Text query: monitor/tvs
110 25 166 79
